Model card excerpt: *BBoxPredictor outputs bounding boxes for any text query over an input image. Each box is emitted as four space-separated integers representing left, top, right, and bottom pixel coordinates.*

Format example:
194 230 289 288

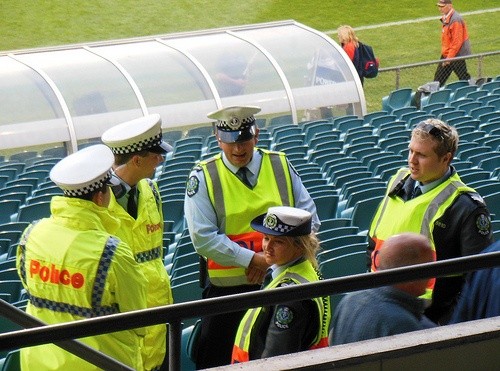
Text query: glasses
417 122 451 152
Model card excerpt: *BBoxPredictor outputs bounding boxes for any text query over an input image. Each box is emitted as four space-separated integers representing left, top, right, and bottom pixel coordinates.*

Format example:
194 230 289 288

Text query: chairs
0 76 500 371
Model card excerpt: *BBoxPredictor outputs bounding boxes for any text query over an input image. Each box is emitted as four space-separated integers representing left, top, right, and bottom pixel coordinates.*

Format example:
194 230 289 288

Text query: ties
412 188 421 199
127 187 137 218
261 271 273 290
239 167 252 188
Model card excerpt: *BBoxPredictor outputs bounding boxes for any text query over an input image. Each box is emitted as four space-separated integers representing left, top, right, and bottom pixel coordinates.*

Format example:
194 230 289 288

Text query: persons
184 105 321 371
366 116 491 327
330 234 437 345
338 25 364 115
232 206 330 362
101 112 174 371
16 144 147 371
214 56 249 97
433 0 473 88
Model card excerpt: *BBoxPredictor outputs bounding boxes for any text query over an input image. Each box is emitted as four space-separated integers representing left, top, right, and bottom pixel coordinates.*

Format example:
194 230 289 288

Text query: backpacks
356 41 379 78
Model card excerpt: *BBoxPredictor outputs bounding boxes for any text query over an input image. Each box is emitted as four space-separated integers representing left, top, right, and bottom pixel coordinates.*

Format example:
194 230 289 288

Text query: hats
206 105 263 142
101 113 174 155
437 0 452 7
249 205 312 236
52 143 121 197
